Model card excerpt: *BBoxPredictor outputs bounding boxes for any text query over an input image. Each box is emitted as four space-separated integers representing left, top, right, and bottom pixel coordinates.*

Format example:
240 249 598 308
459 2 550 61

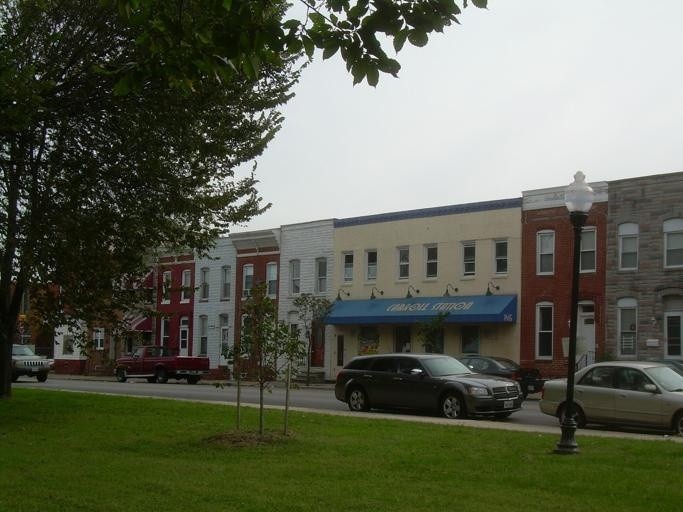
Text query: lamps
486 282 500 296
370 287 383 301
406 285 420 299
443 283 458 297
335 289 350 302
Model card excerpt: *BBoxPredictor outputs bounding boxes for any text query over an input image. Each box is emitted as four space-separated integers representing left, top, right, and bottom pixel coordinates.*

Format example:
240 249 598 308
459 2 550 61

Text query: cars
538 360 683 437
453 353 545 400
644 357 683 371
11 343 54 383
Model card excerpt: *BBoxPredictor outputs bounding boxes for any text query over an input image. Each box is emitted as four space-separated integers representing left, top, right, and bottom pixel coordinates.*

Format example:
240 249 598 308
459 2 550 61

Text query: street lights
553 170 594 455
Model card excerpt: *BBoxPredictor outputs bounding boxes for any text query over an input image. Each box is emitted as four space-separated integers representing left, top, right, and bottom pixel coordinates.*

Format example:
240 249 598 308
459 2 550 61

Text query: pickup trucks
112 345 209 384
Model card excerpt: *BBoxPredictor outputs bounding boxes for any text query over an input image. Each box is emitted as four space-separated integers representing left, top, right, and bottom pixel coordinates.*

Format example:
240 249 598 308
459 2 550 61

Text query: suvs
335 353 524 419
